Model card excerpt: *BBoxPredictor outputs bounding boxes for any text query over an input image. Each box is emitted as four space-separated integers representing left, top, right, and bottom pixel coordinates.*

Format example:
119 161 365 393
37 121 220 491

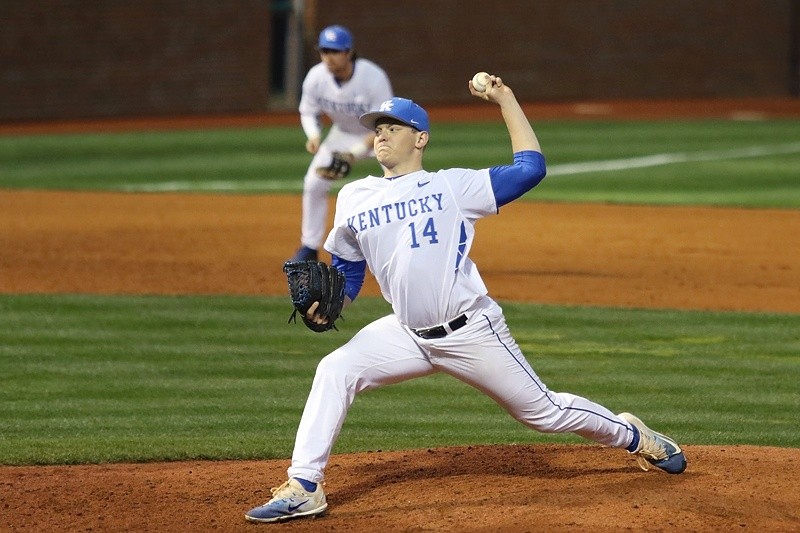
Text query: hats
359 96 430 133
318 24 352 51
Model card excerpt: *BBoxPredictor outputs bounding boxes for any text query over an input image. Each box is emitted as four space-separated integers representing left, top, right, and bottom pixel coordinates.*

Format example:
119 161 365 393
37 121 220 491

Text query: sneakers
245 478 329 524
618 411 687 474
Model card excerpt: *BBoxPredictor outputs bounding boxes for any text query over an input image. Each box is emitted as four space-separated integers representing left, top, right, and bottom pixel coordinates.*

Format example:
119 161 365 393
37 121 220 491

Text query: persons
242 76 686 523
293 26 392 274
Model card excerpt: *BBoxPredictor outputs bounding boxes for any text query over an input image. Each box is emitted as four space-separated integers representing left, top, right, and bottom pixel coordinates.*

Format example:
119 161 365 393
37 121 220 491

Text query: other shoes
288 246 318 261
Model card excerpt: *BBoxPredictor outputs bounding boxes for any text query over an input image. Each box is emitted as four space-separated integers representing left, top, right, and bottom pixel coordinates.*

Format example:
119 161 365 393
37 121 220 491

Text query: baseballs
472 72 491 91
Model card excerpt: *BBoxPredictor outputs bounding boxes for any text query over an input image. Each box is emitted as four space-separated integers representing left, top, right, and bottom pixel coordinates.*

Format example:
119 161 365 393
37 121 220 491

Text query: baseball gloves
284 260 346 333
316 151 354 180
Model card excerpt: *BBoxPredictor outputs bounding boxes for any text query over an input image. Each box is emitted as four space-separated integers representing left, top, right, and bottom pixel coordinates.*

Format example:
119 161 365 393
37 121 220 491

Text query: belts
412 314 468 339
